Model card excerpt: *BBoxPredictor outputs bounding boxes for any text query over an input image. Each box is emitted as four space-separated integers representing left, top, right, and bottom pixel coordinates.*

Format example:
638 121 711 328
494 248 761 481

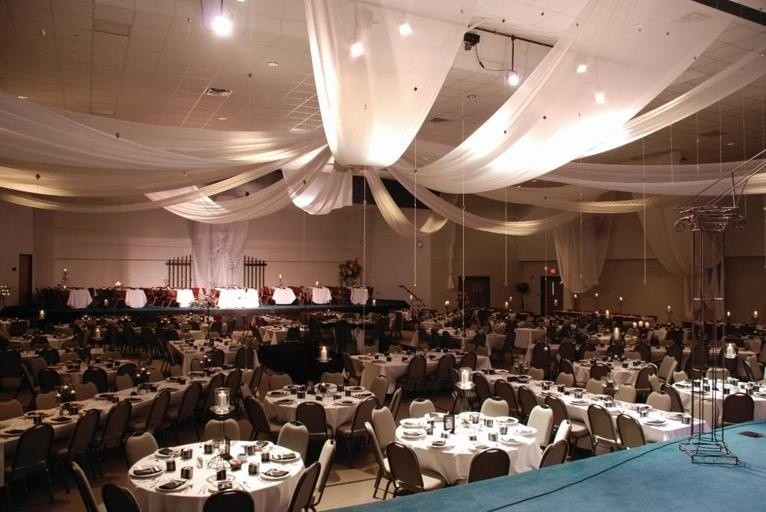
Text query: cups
155 439 273 480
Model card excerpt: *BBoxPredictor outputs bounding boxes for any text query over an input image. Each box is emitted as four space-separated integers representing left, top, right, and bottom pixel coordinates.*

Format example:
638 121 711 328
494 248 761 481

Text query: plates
271 451 301 462
261 472 290 480
155 479 192 491
265 349 766 446
128 465 162 478
2 336 230 436
208 482 239 492
